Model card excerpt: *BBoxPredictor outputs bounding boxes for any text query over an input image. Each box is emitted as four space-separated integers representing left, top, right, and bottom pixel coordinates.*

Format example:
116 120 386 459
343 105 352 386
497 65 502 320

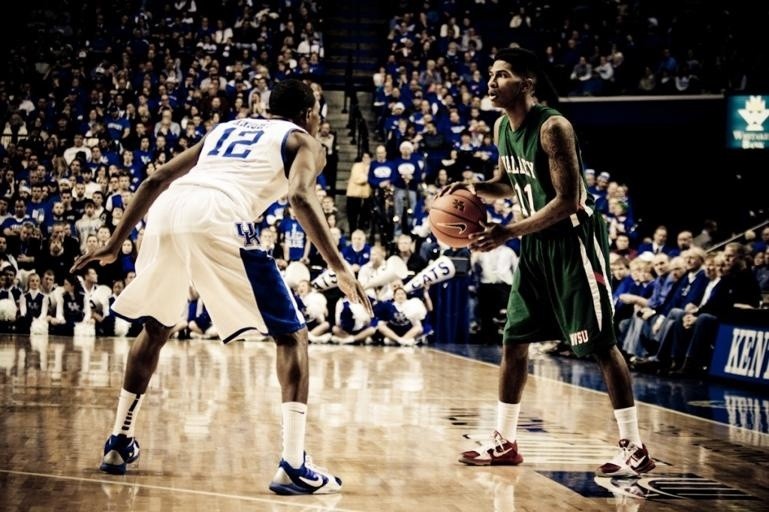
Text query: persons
67 78 377 498
0 0 769 385
430 46 656 478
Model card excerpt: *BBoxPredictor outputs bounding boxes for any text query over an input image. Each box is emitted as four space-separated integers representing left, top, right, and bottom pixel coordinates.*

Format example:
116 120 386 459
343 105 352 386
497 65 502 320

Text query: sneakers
458 432 523 465
100 432 141 476
592 476 645 500
596 438 656 476
268 450 344 495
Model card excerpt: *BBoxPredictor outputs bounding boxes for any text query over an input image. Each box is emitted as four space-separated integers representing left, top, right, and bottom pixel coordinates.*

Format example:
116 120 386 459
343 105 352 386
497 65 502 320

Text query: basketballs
429 189 487 248
